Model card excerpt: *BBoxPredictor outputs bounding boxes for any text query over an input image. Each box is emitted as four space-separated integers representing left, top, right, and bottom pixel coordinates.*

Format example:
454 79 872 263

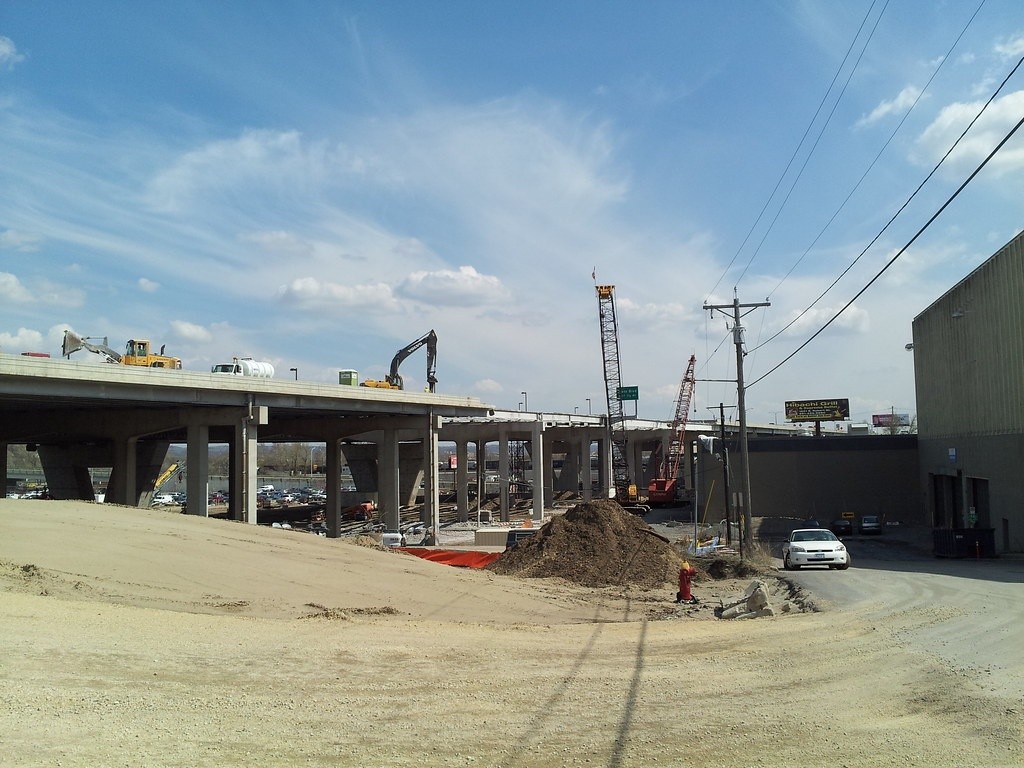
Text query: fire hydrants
678 564 696 601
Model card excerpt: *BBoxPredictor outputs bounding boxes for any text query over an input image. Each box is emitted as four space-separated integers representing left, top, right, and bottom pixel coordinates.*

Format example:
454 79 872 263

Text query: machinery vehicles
212 357 274 378
360 330 439 392
595 284 653 516
647 353 698 508
61 330 182 371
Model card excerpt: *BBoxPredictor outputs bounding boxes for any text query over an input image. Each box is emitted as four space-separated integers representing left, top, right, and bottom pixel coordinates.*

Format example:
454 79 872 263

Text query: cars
153 491 229 504
257 484 357 503
861 515 882 532
830 520 853 536
782 529 851 570
7 491 43 499
801 520 821 529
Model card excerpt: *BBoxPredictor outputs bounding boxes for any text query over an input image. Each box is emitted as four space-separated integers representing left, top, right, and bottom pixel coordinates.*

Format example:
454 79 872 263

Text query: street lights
585 399 591 415
310 447 316 476
575 406 579 413
521 391 527 412
291 368 299 379
519 403 523 410
